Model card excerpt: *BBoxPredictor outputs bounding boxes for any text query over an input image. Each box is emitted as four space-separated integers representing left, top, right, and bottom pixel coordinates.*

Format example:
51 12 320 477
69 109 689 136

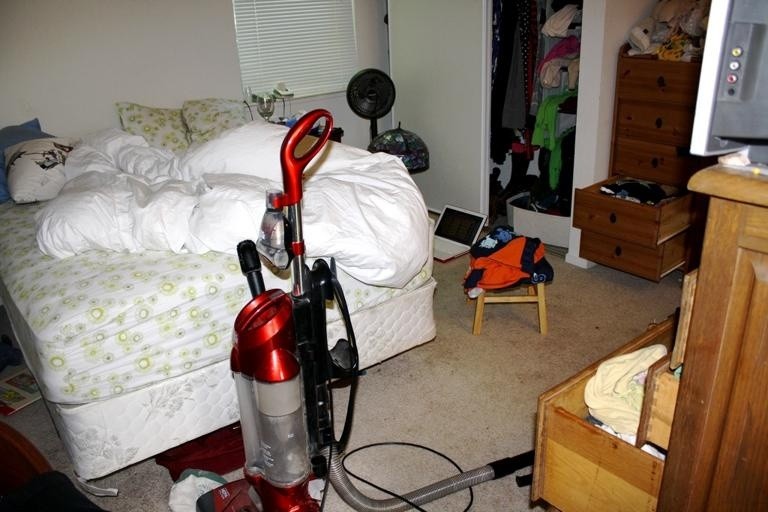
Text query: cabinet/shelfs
385 0 655 271
526 162 767 512
568 40 700 286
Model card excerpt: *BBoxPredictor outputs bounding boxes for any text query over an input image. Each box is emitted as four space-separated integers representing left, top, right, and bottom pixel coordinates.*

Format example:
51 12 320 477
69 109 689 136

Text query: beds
0 120 437 482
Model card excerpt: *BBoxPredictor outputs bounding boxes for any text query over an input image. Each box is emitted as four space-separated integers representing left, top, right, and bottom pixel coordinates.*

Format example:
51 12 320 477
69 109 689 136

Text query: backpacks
463 225 553 293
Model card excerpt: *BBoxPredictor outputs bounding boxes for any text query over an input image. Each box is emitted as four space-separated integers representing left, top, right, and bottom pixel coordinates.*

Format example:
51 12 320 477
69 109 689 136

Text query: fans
343 67 396 140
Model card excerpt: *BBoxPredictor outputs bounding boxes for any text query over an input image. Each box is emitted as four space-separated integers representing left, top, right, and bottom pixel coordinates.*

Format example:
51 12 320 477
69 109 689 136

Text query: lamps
367 118 431 176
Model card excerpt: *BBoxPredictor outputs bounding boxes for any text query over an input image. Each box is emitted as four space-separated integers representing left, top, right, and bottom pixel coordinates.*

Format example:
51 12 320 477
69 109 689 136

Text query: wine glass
256 91 276 122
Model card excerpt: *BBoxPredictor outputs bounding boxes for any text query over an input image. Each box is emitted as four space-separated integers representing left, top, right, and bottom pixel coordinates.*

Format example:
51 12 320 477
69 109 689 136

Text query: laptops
433 204 487 263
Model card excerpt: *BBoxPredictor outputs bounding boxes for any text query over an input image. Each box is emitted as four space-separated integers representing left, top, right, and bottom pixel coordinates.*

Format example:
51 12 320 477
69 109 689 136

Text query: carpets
1 304 175 511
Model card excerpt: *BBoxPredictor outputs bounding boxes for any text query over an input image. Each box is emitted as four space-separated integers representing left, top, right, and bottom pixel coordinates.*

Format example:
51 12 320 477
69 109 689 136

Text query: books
0 367 42 416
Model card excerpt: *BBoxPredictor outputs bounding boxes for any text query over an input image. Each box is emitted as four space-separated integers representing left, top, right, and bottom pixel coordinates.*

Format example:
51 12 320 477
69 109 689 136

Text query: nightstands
309 125 342 142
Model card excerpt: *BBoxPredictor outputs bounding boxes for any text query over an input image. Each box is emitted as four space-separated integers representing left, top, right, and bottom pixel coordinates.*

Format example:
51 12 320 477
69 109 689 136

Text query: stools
467 275 547 338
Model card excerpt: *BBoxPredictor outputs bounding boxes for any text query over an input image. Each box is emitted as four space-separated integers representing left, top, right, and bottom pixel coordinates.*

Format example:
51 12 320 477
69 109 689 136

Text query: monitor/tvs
690 0 768 174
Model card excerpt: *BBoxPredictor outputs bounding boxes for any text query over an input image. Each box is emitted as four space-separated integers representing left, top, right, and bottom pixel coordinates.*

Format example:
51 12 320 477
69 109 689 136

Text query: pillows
180 92 250 144
0 113 58 206
113 99 188 151
3 133 85 205
